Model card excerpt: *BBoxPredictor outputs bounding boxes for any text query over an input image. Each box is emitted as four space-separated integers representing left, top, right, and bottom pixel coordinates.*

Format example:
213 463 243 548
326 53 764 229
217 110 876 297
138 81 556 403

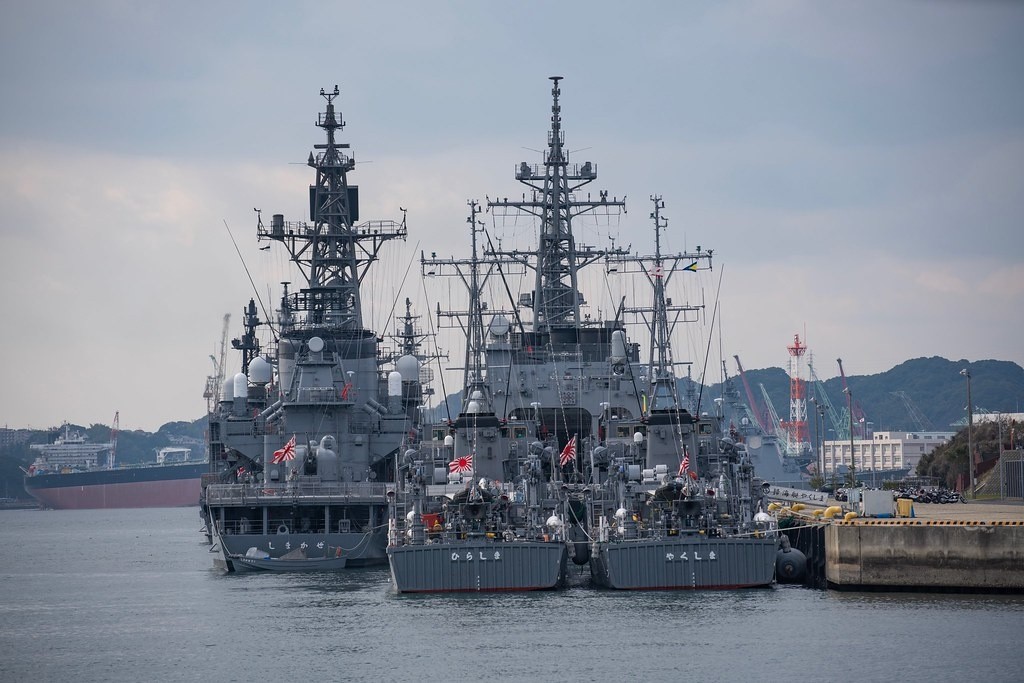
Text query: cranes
731 353 937 461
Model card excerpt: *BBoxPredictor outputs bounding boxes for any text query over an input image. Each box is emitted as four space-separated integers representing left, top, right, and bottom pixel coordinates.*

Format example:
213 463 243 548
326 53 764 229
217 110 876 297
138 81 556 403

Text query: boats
198 69 784 598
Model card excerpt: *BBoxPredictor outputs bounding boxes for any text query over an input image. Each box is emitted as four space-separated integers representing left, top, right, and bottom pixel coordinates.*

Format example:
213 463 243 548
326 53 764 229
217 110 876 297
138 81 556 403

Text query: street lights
842 386 857 489
959 368 975 500
817 404 831 482
809 396 821 477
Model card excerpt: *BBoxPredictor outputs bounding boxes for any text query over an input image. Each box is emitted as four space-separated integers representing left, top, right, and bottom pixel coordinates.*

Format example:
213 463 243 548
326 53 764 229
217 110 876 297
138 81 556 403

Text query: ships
18 429 210 511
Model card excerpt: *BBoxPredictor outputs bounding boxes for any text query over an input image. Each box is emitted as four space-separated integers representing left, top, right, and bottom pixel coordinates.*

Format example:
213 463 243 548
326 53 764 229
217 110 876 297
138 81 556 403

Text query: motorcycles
834 482 970 504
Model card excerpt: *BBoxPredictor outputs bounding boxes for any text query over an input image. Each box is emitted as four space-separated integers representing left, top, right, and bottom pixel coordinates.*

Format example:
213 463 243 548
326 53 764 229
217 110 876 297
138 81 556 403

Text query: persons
291 467 298 480
456 524 465 540
492 523 498 543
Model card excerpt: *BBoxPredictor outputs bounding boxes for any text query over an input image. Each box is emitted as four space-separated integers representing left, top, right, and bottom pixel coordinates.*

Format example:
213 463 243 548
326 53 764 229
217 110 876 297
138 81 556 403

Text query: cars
815 483 844 494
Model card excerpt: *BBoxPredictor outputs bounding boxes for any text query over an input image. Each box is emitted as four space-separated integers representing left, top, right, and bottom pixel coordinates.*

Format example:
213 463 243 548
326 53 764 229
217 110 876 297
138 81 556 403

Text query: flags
271 436 297 464
678 453 690 476
449 454 474 473
237 466 246 476
648 266 664 277
559 436 576 466
